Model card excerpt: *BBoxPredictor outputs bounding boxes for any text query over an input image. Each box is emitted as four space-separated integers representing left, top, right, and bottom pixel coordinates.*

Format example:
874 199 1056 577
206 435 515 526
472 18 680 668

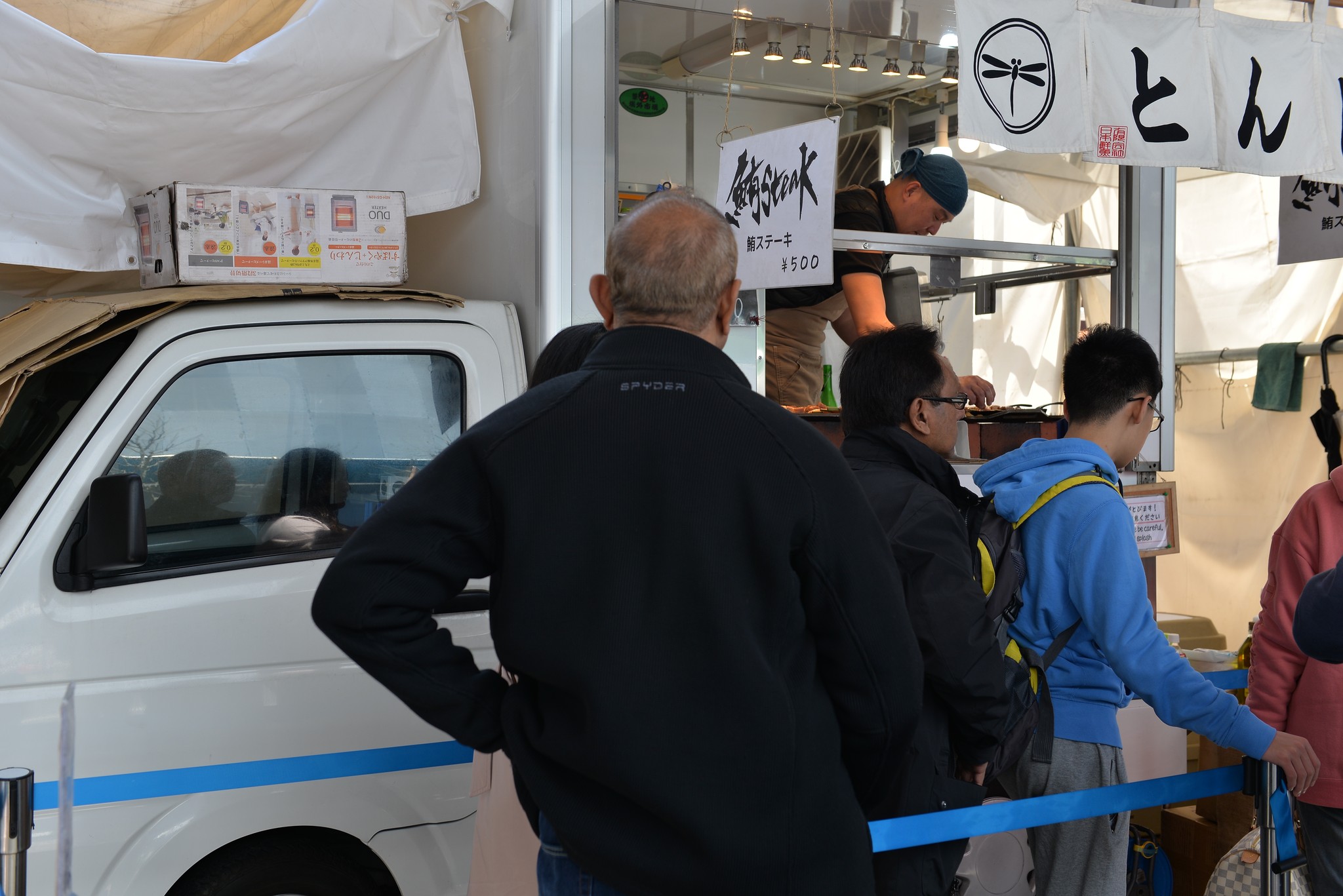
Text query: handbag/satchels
1204 825 1313 896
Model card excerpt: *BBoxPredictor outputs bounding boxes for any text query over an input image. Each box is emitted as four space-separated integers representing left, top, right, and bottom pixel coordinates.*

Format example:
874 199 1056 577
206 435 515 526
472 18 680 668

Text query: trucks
0 0 1179 896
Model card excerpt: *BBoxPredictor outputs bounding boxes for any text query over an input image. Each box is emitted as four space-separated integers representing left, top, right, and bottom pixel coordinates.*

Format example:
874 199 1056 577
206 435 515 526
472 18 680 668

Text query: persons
1244 410 1343 896
763 154 996 410
972 326 1321 896
145 449 256 558
465 323 606 896
309 189 927 895
836 323 1041 895
1292 556 1343 664
252 448 358 555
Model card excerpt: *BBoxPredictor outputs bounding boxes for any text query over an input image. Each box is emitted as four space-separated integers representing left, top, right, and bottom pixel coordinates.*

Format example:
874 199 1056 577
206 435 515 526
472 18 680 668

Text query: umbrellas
1310 334 1343 480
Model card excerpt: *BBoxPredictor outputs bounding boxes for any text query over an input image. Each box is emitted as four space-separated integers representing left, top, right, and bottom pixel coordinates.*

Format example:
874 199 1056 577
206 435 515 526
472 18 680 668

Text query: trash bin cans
1156 612 1227 810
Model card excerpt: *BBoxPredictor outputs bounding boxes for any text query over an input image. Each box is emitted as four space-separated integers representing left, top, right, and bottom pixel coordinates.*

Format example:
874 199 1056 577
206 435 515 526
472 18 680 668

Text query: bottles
1237 621 1255 706
820 364 837 408
1166 632 1186 659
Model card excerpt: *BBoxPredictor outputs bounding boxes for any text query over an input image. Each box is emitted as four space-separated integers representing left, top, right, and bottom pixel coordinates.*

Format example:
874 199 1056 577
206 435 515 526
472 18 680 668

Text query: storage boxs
128 183 409 290
1161 734 1255 896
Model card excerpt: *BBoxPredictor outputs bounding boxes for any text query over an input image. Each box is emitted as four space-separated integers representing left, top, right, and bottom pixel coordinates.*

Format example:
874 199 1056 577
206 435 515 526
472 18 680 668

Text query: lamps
821 30 841 68
763 23 784 61
940 48 958 84
907 43 927 79
792 27 812 64
731 18 750 56
882 40 901 76
848 35 868 72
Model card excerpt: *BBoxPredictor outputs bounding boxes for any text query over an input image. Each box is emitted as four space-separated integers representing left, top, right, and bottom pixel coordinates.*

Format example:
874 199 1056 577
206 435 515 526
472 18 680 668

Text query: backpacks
977 470 1126 787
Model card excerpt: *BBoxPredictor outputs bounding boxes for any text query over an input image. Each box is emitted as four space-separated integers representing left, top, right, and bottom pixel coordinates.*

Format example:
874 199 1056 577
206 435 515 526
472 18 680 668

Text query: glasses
1128 396 1165 433
909 391 968 410
